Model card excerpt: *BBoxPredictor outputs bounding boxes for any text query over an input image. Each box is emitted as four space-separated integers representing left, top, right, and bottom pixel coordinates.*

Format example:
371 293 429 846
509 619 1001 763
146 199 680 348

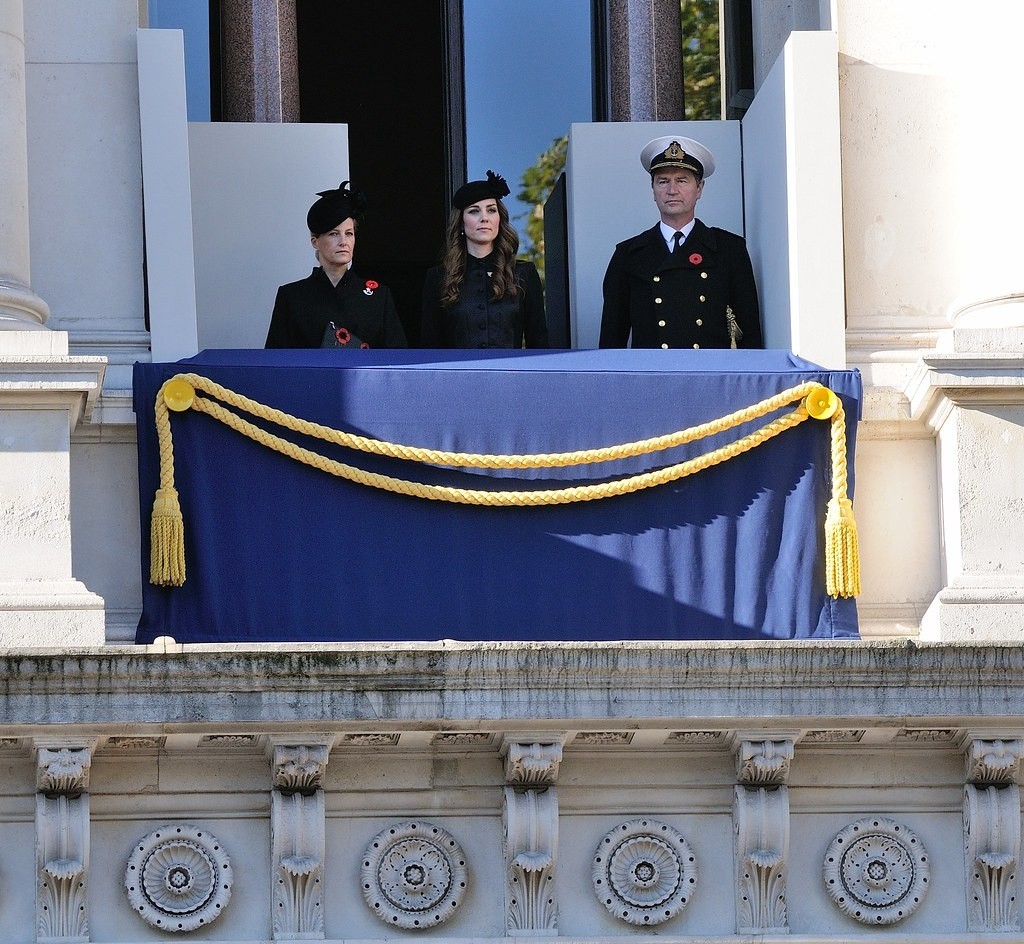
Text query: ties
672 232 683 253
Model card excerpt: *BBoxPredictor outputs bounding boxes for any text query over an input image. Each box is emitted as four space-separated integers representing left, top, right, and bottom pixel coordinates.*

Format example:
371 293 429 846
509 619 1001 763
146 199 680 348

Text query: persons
420 170 549 349
265 180 408 349
600 135 762 349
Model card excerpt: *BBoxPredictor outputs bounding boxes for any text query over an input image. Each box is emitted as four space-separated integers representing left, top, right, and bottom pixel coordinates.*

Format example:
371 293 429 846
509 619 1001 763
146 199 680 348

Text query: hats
307 181 357 236
640 136 715 180
453 171 510 210
